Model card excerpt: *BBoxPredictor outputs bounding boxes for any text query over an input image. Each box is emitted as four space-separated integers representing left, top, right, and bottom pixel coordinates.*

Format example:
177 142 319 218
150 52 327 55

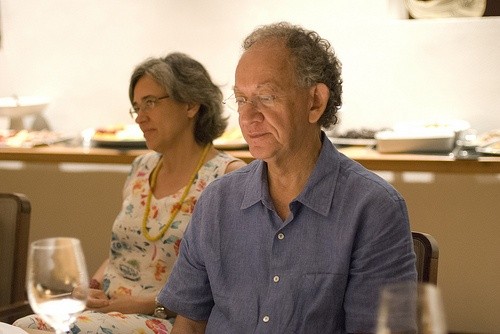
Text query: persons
157 21 418 334
12 52 248 334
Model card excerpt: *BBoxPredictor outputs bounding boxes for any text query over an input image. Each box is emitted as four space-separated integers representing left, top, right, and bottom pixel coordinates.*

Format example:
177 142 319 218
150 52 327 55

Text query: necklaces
141 142 211 243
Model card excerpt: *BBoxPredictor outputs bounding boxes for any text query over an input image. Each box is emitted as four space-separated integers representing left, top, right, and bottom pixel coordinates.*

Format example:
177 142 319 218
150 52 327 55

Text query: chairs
0 191 32 324
411 229 440 288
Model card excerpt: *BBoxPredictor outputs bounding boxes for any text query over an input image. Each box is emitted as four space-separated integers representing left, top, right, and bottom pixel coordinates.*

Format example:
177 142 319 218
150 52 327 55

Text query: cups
25 236 89 334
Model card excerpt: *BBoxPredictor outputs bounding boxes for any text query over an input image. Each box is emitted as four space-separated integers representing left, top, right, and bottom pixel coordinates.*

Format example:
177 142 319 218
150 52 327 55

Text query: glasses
223 88 311 111
129 96 168 119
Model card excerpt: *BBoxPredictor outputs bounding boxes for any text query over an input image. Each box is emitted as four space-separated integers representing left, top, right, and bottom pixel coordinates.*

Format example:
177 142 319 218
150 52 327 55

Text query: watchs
152 296 168 319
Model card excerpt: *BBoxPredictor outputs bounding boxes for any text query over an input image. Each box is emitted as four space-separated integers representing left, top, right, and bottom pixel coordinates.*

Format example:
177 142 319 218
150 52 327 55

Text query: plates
213 139 247 149
89 138 146 145
